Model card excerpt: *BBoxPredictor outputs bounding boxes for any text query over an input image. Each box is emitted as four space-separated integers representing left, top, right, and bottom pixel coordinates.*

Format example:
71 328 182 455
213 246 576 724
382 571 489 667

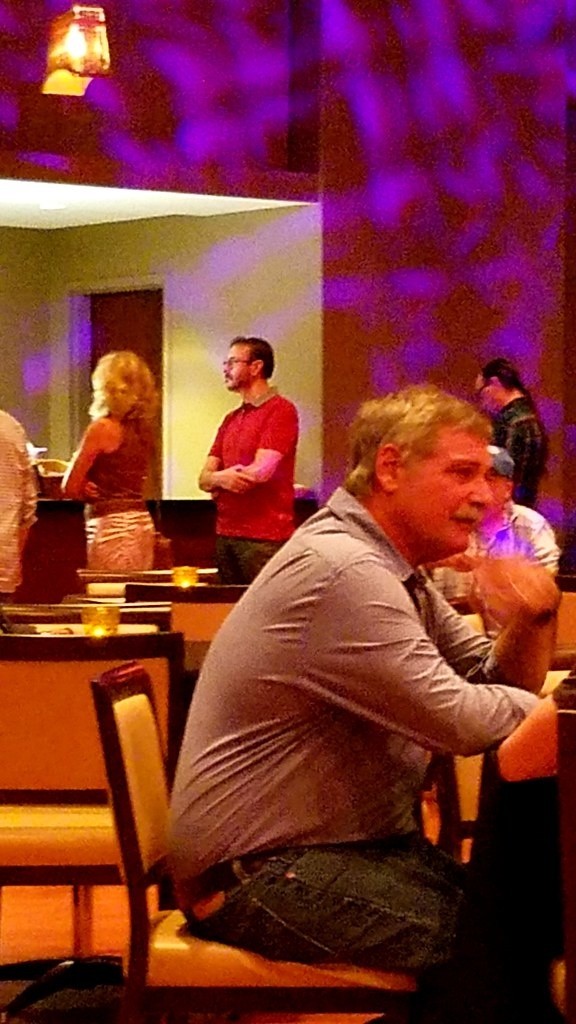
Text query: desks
469 668 576 1024
0 622 157 636
76 595 126 606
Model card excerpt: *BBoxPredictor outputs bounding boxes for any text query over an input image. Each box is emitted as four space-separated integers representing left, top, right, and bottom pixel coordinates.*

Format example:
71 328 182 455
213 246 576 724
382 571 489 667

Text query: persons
421 448 562 601
496 664 576 783
62 349 158 575
199 334 300 585
0 410 43 605
160 382 570 1024
475 357 545 510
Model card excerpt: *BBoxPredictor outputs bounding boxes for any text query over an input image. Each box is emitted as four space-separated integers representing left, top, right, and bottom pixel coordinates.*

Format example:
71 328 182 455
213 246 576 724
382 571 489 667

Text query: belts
172 847 294 909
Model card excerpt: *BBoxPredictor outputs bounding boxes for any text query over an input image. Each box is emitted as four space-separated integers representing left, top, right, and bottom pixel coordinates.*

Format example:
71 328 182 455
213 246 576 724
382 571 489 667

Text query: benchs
0 629 185 911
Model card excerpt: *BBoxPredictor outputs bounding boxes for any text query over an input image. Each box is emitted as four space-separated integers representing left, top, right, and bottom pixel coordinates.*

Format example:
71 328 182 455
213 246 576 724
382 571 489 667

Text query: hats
485 445 512 476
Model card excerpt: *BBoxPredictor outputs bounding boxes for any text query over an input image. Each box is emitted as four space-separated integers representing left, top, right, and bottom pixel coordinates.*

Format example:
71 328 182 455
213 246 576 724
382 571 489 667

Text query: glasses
474 383 489 397
225 358 251 367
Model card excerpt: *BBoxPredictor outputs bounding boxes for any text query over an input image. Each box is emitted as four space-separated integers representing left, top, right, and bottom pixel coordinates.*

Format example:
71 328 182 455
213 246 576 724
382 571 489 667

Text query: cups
81 606 122 637
173 565 198 586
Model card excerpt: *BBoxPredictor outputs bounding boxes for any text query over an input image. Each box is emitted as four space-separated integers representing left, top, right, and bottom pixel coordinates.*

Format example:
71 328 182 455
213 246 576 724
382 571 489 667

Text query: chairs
82 573 136 599
90 663 430 1024
135 566 221 583
551 593 576 670
433 601 486 862
124 583 250 681
